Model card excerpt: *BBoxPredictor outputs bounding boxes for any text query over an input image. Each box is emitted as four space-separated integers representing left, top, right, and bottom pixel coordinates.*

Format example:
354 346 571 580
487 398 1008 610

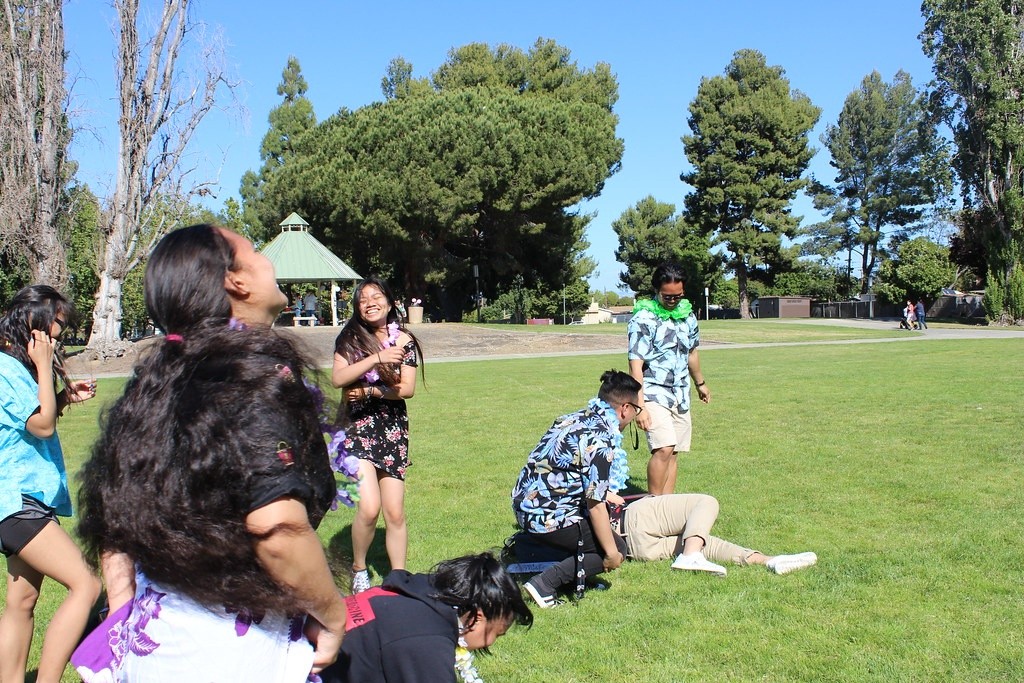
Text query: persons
500 493 817 578
628 265 710 497
395 299 405 322
0 285 103 683
70 224 347 683
294 289 317 325
511 368 644 609
331 279 426 591
906 300 915 330
318 550 534 683
913 298 927 330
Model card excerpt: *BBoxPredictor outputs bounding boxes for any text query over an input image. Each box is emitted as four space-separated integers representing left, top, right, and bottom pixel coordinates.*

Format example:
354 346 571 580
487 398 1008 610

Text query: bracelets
366 386 387 402
694 380 705 386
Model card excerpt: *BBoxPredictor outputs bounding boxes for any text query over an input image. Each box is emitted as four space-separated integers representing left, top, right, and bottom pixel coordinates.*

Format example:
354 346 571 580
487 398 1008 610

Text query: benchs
293 317 317 326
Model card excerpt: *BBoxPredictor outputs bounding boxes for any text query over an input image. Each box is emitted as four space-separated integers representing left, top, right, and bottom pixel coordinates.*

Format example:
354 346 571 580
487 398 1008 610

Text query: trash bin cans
611 318 617 324
408 307 423 324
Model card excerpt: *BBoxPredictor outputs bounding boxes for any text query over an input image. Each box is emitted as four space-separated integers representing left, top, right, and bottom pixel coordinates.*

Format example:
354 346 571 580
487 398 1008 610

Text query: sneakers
671 552 727 578
766 552 817 575
524 573 559 610
350 568 370 596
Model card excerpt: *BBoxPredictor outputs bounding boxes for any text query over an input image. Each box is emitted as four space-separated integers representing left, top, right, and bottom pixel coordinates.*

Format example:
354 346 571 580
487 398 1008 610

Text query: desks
281 310 321 325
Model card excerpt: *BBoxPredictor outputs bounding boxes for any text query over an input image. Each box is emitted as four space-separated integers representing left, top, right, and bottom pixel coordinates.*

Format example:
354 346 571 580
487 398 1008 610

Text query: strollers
900 317 918 329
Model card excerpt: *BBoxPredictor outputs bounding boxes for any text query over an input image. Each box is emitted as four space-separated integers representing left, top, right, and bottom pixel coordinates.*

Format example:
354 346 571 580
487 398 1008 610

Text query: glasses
622 401 643 416
659 290 684 303
52 316 71 336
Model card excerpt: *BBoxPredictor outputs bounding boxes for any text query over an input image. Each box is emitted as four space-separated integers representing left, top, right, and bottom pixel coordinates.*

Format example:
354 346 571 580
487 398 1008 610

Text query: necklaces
229 319 362 508
455 616 482 683
353 322 399 382
633 297 692 321
